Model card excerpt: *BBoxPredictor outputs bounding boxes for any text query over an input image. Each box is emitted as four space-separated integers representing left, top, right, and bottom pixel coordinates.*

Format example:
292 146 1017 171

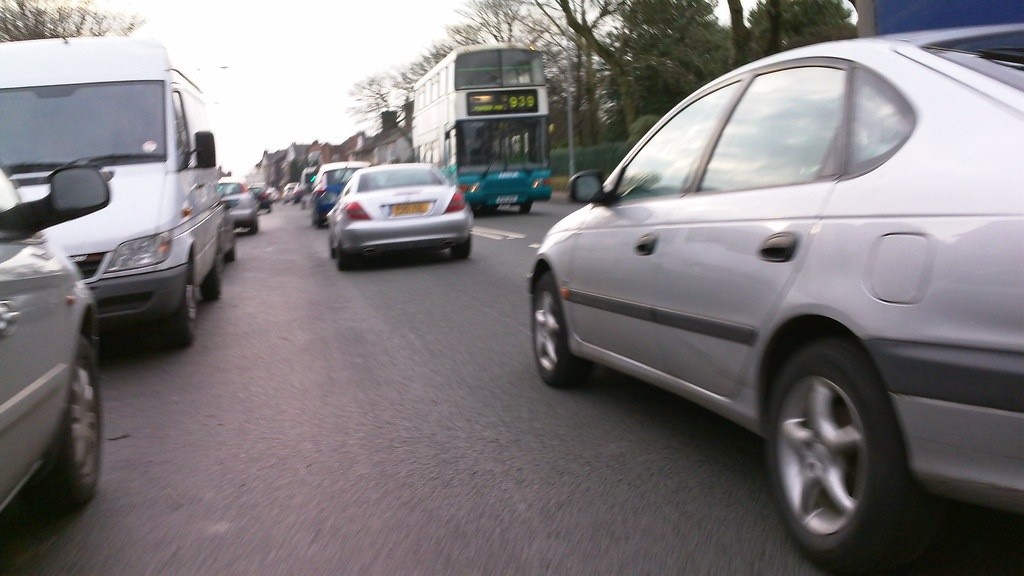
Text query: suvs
282 159 372 227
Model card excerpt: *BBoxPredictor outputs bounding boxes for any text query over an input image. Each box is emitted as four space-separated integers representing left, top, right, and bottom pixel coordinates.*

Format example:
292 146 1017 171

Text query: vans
0 37 235 349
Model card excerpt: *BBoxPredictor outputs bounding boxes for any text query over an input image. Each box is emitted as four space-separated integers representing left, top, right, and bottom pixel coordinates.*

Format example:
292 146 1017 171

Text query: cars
527 23 1024 576
218 177 279 233
0 166 114 513
326 162 475 272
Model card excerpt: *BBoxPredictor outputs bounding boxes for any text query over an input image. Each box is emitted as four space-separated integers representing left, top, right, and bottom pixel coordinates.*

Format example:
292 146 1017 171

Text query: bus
381 43 552 215
